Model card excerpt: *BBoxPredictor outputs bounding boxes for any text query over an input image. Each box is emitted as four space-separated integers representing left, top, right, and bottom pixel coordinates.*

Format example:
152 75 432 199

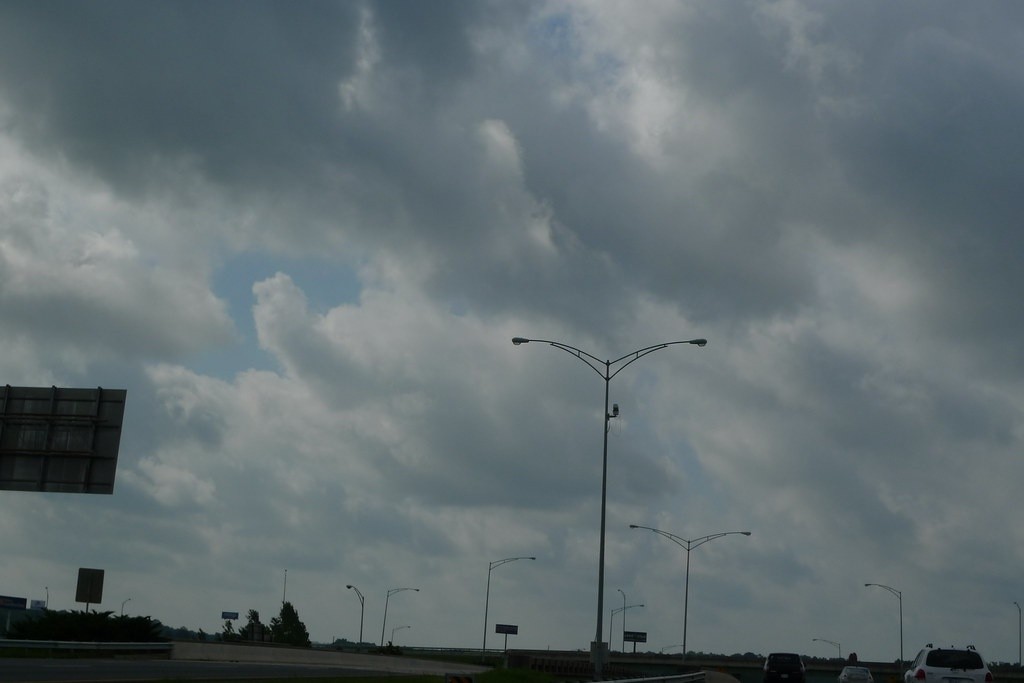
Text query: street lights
346 584 365 644
510 334 708 683
864 582 903 680
1013 602 1022 667
121 597 132 617
381 588 420 647
629 524 752 664
617 588 626 657
811 638 841 666
480 556 536 662
44 586 51 610
391 625 411 644
608 603 645 653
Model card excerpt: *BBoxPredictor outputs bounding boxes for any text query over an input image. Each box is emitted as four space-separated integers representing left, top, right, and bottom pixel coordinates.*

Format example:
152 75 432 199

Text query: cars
837 665 874 683
903 643 994 683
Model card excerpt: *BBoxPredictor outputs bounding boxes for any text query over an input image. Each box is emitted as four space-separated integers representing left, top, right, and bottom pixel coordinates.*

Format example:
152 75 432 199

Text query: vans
762 652 810 683
